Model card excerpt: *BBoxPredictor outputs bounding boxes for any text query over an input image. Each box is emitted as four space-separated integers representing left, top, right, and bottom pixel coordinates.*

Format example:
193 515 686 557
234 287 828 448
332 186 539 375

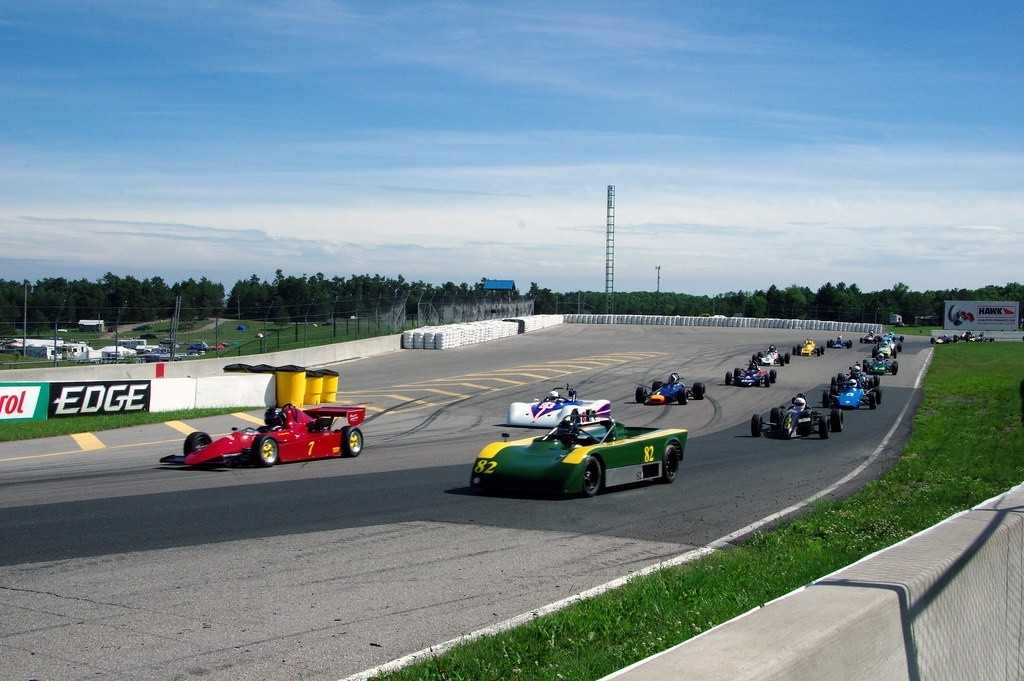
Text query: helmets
849 379 857 388
883 341 888 347
769 345 776 352
668 373 680 383
838 336 842 340
878 353 884 361
853 366 861 374
794 398 806 411
548 390 558 401
264 407 284 425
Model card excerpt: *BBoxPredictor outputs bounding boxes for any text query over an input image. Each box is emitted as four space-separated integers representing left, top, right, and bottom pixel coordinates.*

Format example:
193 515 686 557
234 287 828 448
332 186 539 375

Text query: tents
237 325 246 331
187 341 238 352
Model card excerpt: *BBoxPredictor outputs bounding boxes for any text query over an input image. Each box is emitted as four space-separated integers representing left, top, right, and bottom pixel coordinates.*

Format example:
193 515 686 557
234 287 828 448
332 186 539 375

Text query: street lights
24 283 33 357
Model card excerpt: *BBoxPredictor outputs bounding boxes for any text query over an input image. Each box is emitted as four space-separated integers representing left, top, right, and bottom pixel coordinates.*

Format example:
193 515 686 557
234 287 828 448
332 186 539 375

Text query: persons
805 337 812 344
889 331 893 336
749 361 757 370
980 332 983 336
878 353 884 362
870 330 873 334
264 406 287 427
837 335 842 341
556 420 583 448
848 379 858 389
547 390 563 403
944 334 947 338
853 365 860 377
769 343 776 352
794 398 806 414
668 375 677 384
970 331 973 335
882 341 889 347
